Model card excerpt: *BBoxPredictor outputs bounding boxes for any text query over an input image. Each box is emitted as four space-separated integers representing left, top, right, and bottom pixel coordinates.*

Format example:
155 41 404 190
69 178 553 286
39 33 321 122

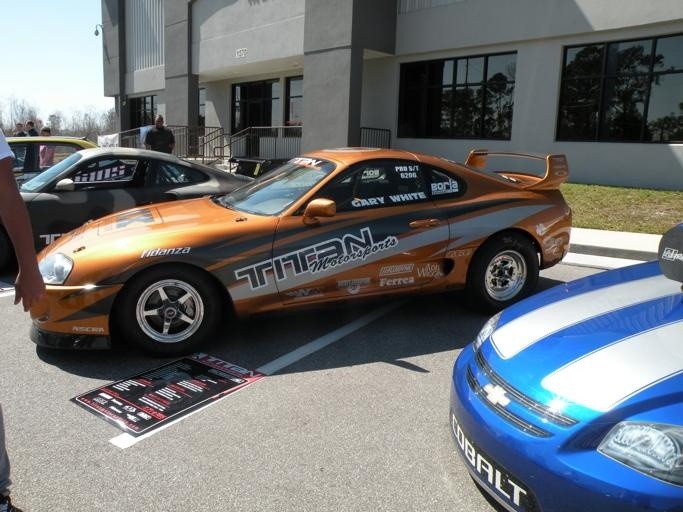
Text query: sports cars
30 151 572 357
450 220 683 510
1 146 250 273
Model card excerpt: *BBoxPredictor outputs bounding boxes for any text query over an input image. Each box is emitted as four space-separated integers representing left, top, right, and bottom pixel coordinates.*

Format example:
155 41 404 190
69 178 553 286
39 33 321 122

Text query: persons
0 128 46 512
143 115 175 154
39 127 54 170
13 124 26 158
24 121 39 171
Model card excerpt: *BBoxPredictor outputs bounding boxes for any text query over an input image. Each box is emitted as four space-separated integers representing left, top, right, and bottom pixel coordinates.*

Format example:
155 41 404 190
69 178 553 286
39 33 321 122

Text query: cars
5 136 94 184
